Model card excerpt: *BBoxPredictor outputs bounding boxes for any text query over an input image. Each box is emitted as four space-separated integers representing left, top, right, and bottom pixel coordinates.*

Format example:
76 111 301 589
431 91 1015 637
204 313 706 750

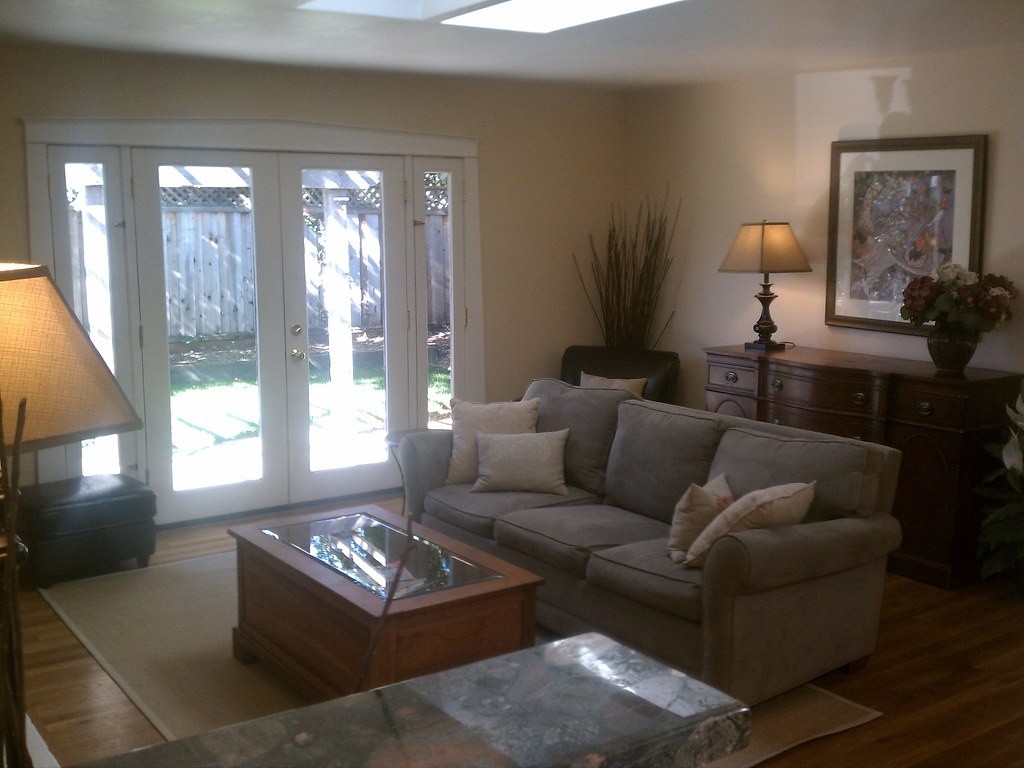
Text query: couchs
397 377 901 708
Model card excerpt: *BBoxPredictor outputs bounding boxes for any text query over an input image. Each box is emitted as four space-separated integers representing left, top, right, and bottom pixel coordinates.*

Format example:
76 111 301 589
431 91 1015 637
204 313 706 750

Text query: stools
9 474 158 587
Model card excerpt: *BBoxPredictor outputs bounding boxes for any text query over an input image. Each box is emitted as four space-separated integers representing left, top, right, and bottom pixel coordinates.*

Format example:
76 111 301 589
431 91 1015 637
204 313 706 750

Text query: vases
927 322 978 372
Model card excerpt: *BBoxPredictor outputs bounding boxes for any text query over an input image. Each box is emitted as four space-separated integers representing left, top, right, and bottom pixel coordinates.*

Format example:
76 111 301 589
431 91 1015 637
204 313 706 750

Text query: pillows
580 370 647 398
472 428 571 496
669 472 733 564
684 481 817 569
442 398 542 487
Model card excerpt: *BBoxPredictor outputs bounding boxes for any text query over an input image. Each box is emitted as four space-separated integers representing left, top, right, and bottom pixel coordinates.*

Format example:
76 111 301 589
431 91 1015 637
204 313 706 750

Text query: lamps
718 219 813 351
0 262 146 717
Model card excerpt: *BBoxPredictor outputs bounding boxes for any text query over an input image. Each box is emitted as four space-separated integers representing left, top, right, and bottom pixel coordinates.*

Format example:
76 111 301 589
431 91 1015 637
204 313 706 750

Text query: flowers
900 263 1021 334
972 382 1024 578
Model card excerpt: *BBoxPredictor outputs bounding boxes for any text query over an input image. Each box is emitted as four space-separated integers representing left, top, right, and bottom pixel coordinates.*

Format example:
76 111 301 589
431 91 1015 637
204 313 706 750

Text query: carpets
39 551 883 768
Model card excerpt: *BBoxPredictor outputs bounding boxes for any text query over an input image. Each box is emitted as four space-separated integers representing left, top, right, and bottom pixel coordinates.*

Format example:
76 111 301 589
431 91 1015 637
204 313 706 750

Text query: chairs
511 344 681 403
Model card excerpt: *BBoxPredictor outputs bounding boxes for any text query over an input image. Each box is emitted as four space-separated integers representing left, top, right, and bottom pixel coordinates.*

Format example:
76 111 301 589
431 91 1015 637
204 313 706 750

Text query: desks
386 428 452 517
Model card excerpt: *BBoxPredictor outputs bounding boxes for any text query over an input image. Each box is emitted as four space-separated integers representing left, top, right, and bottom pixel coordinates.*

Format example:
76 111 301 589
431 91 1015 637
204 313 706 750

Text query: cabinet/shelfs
705 343 1024 589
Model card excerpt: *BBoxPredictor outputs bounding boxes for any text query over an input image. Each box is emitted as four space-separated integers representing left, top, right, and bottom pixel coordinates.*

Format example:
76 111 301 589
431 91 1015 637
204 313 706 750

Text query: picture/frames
825 135 988 338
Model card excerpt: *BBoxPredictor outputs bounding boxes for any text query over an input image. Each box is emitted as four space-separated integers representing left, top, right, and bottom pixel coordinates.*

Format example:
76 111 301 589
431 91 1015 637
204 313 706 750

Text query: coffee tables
226 506 548 703
